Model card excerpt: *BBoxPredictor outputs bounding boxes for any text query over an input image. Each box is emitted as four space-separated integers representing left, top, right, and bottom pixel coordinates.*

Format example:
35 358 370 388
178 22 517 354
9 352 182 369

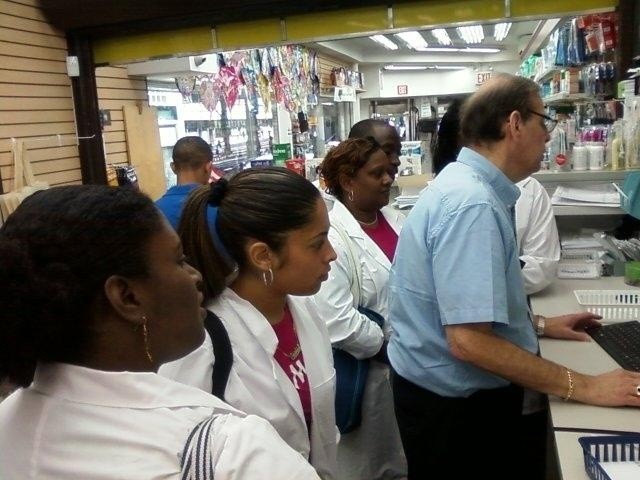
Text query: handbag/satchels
330 304 384 436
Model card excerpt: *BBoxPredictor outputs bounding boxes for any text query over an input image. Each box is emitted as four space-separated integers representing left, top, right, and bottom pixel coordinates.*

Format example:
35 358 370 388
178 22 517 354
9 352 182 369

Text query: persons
157 167 341 479
431 97 561 480
310 137 408 480
155 135 236 270
348 119 402 181
0 185 319 480
387 72 640 480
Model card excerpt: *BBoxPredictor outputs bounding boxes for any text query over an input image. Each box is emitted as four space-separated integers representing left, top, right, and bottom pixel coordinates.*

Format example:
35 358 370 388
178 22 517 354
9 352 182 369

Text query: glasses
524 109 559 133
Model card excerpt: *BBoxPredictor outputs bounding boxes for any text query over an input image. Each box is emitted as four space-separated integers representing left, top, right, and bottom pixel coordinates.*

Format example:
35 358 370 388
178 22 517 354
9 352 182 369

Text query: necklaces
357 211 378 225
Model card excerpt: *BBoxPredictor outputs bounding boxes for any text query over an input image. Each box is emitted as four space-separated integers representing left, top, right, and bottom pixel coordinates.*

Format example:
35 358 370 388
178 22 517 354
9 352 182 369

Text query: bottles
572 129 625 171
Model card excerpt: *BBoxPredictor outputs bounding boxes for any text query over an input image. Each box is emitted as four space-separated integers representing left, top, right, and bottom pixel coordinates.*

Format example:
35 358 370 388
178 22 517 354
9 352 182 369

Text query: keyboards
584 320 640 372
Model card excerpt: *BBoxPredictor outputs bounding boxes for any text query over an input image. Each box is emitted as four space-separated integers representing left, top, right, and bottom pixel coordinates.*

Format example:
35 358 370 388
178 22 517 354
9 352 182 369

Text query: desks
529 274 639 480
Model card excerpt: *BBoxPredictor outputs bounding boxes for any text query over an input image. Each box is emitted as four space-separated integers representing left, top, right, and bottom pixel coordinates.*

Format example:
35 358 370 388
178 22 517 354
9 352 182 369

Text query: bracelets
563 366 572 401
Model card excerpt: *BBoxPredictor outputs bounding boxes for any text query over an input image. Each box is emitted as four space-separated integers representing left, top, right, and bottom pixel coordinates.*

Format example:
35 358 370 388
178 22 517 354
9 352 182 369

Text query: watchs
535 314 546 337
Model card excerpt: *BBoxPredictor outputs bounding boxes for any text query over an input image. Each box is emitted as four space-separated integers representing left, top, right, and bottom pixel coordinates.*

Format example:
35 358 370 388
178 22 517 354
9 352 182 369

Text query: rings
636 386 640 397
